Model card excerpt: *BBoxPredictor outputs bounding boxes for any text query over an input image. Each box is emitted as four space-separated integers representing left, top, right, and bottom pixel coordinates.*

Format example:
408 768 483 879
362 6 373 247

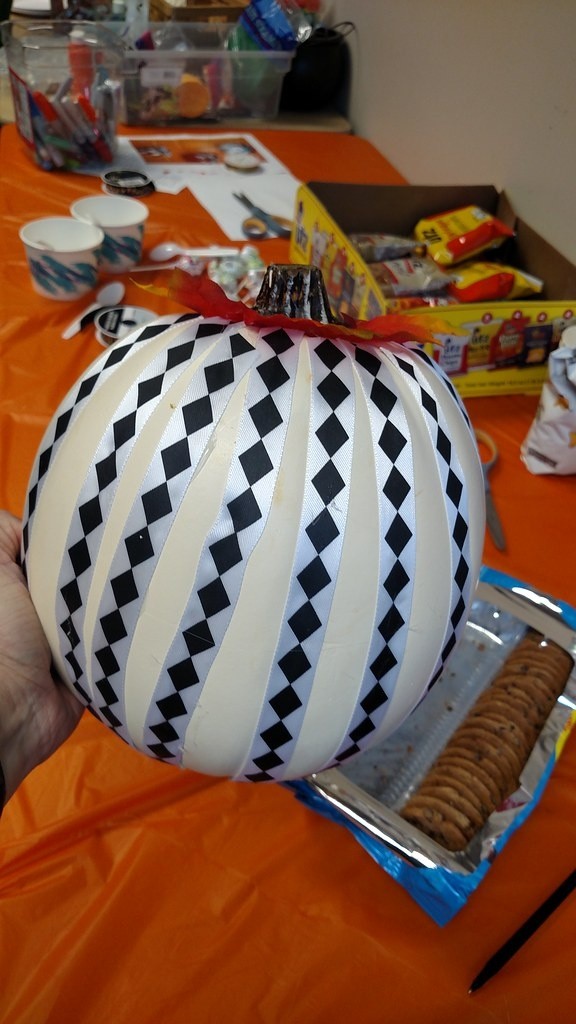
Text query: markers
27 70 114 173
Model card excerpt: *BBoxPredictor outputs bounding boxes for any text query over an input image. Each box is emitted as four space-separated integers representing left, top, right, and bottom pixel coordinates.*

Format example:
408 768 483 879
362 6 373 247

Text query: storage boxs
288 180 576 401
121 22 296 127
0 19 125 173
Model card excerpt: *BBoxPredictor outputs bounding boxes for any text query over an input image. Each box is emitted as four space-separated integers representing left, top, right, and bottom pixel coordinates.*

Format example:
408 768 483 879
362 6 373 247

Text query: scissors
231 190 292 239
469 430 508 556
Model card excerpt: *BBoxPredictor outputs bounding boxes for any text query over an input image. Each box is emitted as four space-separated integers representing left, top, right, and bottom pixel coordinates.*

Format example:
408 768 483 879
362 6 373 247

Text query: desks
0 120 576 1024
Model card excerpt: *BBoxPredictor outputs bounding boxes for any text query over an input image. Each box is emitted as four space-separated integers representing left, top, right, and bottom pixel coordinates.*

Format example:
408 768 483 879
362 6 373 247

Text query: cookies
399 633 573 852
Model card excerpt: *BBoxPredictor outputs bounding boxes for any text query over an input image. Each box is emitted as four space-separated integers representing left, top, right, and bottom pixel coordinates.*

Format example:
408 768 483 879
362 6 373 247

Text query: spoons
62 282 125 340
150 244 239 261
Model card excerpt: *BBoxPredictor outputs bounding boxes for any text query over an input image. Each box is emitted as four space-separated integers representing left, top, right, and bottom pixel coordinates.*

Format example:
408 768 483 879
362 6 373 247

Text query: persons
0 509 84 815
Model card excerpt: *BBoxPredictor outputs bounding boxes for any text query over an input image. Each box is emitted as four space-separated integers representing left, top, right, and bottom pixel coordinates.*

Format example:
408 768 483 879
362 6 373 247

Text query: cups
19 217 104 301
70 194 149 273
